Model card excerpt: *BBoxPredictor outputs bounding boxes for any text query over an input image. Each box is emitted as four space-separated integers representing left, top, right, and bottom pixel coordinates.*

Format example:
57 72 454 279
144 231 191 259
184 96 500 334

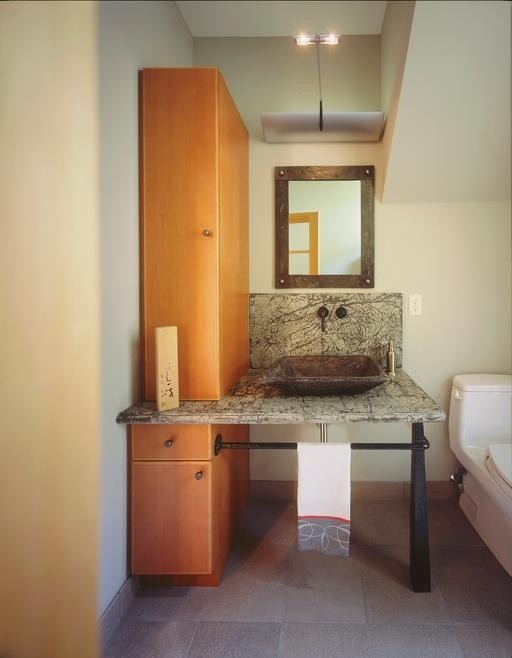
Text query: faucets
317 307 330 332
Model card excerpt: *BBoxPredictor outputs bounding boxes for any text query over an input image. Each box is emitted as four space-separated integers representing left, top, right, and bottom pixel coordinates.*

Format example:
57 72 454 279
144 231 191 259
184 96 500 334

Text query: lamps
296 34 342 47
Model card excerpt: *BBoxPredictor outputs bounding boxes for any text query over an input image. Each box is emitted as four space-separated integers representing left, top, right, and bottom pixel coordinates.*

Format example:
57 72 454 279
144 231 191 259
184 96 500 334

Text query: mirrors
274 166 375 288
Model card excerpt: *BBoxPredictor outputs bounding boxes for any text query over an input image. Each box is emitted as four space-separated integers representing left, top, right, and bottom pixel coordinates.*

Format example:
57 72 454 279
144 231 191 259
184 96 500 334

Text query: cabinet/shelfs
139 67 250 402
129 424 250 587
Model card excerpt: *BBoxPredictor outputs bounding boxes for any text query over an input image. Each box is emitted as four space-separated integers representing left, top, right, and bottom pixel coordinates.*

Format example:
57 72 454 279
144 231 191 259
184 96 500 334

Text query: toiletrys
386 339 396 374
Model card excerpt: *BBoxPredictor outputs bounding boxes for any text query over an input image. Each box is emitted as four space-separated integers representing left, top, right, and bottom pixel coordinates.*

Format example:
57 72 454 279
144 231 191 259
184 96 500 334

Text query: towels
297 443 351 558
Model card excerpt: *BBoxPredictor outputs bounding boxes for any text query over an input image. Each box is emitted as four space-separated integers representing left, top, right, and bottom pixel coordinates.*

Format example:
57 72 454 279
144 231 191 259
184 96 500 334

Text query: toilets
448 374 512 577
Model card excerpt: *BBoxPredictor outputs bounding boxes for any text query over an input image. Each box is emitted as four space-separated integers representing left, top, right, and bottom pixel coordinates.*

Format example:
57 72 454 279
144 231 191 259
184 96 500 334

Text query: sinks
260 356 390 395
